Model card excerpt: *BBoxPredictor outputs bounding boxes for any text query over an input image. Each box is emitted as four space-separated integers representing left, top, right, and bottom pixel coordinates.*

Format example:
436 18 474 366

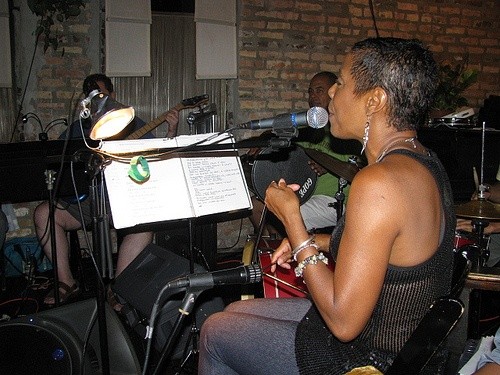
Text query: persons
42 73 180 311
199 36 500 375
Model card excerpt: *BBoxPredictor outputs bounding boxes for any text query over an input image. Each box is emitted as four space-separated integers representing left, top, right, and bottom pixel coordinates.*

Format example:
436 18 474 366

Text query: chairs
343 243 481 375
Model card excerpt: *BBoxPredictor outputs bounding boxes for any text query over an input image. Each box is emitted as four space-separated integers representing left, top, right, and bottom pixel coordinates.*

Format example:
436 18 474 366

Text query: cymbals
455 199 500 219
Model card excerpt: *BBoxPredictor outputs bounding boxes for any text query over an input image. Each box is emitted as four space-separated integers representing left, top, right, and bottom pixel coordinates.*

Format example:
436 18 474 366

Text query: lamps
81 77 135 141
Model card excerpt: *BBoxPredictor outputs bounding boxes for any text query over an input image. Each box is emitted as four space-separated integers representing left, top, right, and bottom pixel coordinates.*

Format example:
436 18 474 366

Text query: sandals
107 290 123 313
43 282 80 308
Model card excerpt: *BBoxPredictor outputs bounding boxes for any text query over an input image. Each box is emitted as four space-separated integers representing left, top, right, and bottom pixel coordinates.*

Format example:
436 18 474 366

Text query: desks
418 126 500 201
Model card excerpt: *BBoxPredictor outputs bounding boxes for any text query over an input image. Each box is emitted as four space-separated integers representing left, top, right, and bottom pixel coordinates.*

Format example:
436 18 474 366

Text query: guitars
61 94 210 205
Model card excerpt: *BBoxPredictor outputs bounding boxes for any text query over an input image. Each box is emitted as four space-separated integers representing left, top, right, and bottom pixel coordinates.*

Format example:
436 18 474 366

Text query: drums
452 230 495 253
243 142 317 206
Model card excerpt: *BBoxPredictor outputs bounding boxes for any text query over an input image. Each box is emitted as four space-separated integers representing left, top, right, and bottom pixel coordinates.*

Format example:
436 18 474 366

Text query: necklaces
385 137 418 151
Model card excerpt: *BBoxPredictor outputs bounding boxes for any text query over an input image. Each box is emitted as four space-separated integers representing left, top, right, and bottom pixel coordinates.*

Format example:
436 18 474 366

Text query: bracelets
294 252 328 277
294 245 317 261
290 233 315 254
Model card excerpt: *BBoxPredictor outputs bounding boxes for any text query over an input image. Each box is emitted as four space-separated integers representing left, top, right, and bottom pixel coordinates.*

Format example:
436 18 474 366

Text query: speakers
0 242 228 375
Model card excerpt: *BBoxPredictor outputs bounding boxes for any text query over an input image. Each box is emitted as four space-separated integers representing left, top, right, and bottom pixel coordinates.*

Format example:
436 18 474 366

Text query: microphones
167 263 262 289
236 106 328 128
